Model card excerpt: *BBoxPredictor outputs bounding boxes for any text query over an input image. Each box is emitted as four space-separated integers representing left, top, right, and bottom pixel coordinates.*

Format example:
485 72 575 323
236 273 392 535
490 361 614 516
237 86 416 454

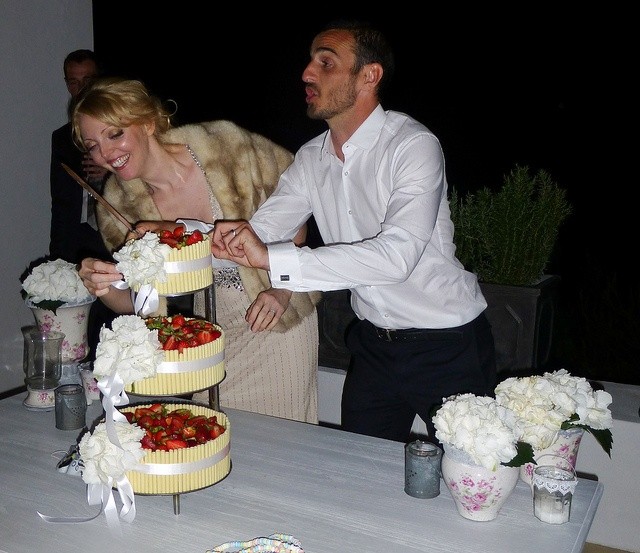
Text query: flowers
494 368 614 459
21 256 97 304
432 392 537 467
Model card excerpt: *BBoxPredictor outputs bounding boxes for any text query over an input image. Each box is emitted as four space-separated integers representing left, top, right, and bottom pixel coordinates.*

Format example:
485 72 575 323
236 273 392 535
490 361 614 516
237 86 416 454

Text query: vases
519 427 586 484
28 304 100 405
440 452 520 522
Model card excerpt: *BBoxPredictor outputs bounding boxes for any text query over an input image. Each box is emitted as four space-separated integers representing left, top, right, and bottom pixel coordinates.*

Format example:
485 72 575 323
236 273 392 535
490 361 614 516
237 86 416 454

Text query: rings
231 229 236 238
267 309 276 314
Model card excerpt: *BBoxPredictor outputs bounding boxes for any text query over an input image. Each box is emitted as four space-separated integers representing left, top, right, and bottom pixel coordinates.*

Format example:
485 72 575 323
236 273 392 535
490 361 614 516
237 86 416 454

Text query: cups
22 329 64 390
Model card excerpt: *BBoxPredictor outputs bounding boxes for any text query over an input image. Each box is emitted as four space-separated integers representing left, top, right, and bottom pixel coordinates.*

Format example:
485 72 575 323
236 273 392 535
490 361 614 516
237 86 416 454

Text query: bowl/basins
22 386 57 409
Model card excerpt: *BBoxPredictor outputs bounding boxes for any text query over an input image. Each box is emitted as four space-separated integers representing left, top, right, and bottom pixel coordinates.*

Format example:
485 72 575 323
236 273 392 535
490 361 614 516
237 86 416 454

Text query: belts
372 328 432 342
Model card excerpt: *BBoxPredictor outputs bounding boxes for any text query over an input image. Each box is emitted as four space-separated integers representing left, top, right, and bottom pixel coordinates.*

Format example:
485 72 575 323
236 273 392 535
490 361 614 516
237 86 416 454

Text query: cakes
97 402 232 494
125 227 214 295
124 313 226 395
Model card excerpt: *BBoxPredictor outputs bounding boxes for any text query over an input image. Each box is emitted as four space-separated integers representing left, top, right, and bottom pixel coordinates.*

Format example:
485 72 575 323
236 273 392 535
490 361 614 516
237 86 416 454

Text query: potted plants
448 160 572 375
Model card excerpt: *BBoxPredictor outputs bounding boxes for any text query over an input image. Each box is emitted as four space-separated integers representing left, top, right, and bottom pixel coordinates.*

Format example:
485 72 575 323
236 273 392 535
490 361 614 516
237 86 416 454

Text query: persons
119 25 498 444
71 78 320 424
48 48 106 267
